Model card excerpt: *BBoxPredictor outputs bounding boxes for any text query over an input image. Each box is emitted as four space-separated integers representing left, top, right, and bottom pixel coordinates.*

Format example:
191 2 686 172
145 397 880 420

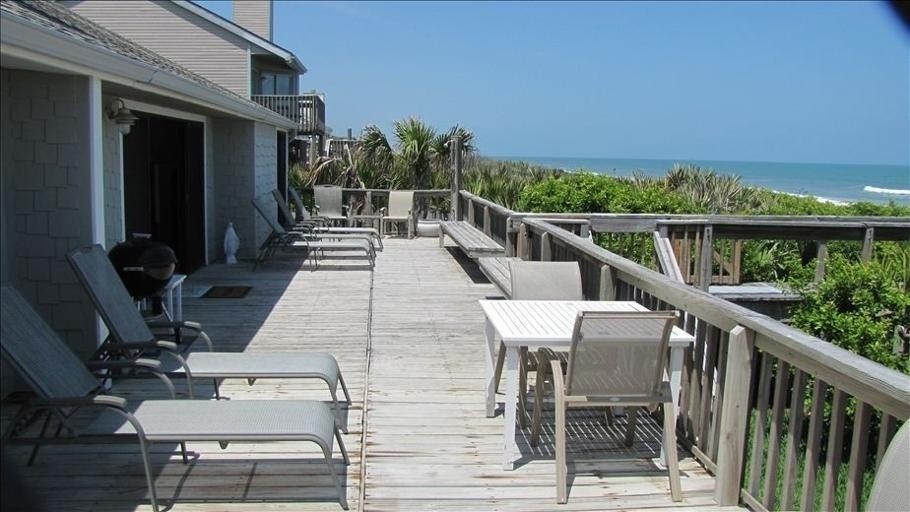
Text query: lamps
104 96 136 137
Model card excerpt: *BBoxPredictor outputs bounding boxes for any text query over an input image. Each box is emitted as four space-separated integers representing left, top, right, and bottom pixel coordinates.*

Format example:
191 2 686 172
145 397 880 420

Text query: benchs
440 220 526 301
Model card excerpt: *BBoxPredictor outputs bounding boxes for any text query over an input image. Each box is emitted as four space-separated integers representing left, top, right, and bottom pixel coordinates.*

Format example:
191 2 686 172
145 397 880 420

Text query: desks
478 300 696 471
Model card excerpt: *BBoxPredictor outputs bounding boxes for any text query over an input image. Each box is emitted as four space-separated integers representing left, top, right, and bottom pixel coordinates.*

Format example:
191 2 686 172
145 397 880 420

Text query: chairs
66 243 351 435
508 260 583 428
1 283 350 512
106 243 187 335
530 309 684 504
250 181 418 271
